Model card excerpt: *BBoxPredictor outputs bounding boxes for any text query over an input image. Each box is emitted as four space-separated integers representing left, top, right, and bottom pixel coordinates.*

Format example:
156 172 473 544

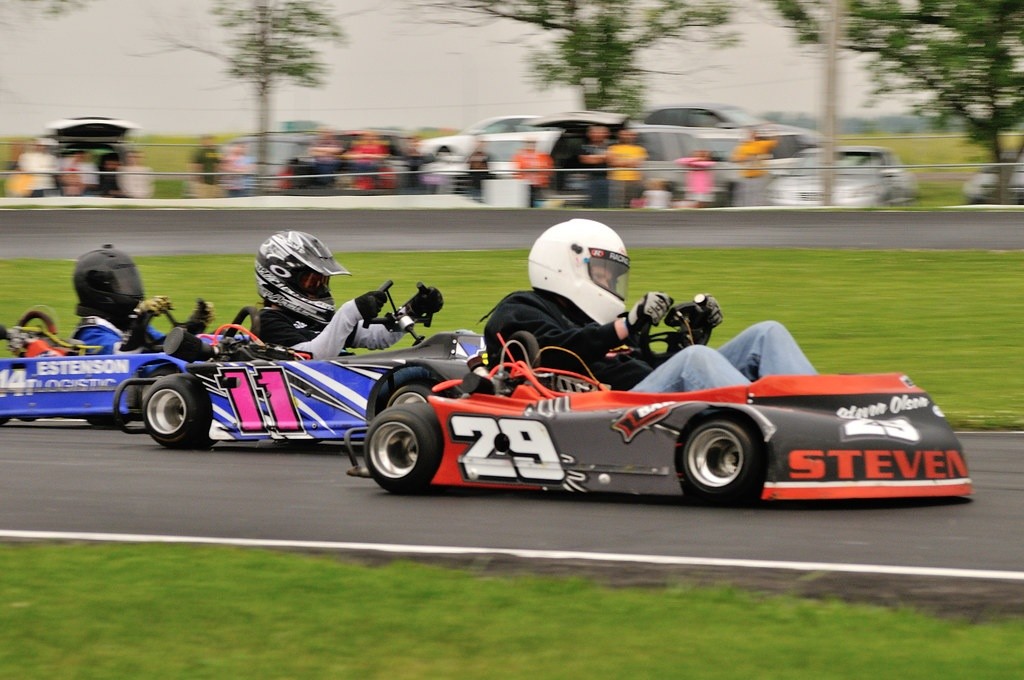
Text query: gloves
419 286 444 313
617 291 674 337
693 294 723 329
134 295 172 317
190 298 214 326
355 291 388 320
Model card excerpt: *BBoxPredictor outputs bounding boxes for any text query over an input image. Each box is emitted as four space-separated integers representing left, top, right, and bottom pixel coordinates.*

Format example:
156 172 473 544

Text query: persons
6 122 779 210
252 228 443 360
67 244 214 355
484 218 819 394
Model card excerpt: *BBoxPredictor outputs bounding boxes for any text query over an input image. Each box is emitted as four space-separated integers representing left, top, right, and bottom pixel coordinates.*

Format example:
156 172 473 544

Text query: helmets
528 218 630 327
74 250 144 324
254 231 354 325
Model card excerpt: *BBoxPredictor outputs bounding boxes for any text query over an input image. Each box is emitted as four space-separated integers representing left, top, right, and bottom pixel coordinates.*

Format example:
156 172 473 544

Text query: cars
962 140 1024 203
633 105 825 152
416 115 566 165
229 134 315 189
38 115 137 197
812 145 920 202
